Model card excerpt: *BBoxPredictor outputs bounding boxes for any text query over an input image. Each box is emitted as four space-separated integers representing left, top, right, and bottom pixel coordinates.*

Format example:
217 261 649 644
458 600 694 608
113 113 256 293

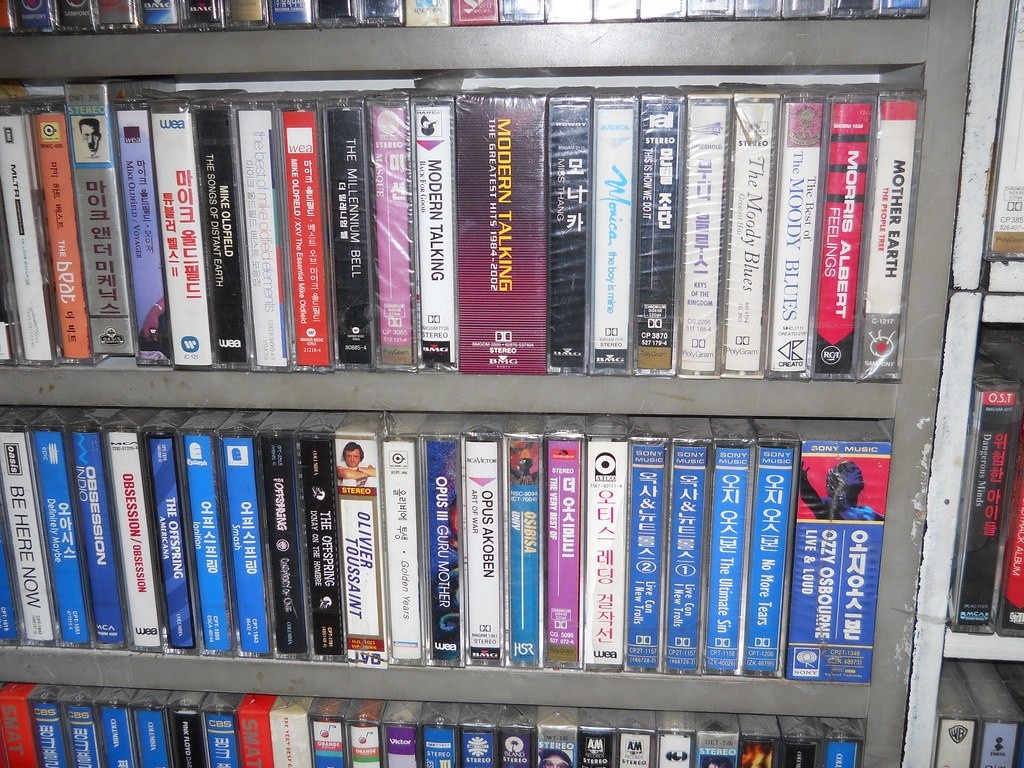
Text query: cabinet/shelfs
0 1 978 768
899 1 1024 768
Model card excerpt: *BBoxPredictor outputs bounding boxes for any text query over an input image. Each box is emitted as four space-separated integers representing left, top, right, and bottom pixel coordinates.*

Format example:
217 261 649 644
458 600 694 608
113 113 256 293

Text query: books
0 405 891 685
0 0 934 33
932 662 1024 768
0 682 867 768
0 79 926 385
992 0 1024 254
949 365 1024 639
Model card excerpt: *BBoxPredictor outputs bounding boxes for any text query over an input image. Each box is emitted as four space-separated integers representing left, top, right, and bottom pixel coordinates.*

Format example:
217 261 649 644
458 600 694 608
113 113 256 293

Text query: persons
537 747 573 768
336 442 376 487
